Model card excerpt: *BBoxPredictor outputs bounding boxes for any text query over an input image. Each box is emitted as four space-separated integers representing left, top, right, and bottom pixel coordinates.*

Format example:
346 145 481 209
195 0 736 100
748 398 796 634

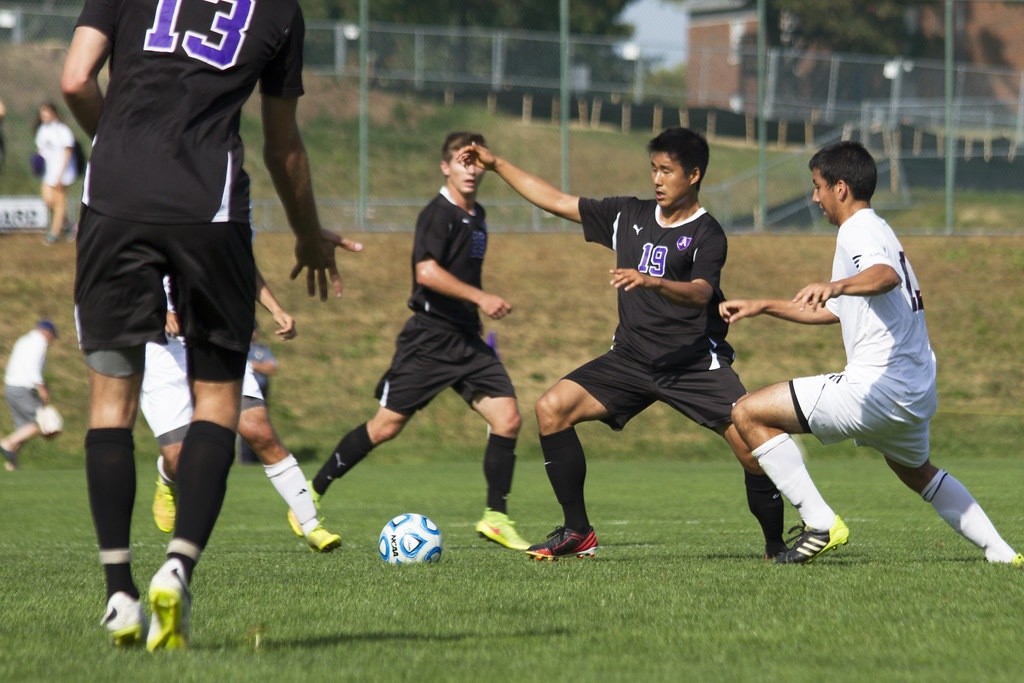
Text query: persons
32 0 363 650
719 141 1024 567
287 131 533 551
73 228 343 552
455 127 790 562
0 322 58 471
32 99 83 248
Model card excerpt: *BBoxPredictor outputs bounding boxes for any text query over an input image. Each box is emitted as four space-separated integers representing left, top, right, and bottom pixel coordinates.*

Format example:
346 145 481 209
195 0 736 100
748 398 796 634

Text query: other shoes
42 222 77 246
1 443 22 472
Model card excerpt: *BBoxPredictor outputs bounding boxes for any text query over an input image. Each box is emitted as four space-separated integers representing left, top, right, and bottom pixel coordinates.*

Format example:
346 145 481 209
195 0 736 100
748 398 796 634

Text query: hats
38 320 60 339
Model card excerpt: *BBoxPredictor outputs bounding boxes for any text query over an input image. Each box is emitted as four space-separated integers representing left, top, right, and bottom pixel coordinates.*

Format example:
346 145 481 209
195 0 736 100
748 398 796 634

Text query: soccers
378 512 443 566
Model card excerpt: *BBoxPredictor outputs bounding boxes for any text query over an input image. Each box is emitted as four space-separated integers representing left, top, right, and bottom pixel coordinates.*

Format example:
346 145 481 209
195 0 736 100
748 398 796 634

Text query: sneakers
304 527 342 552
770 516 849 564
525 526 600 562
146 557 193 650
476 509 531 551
153 471 177 533
102 590 146 646
764 536 788 561
287 481 317 535
